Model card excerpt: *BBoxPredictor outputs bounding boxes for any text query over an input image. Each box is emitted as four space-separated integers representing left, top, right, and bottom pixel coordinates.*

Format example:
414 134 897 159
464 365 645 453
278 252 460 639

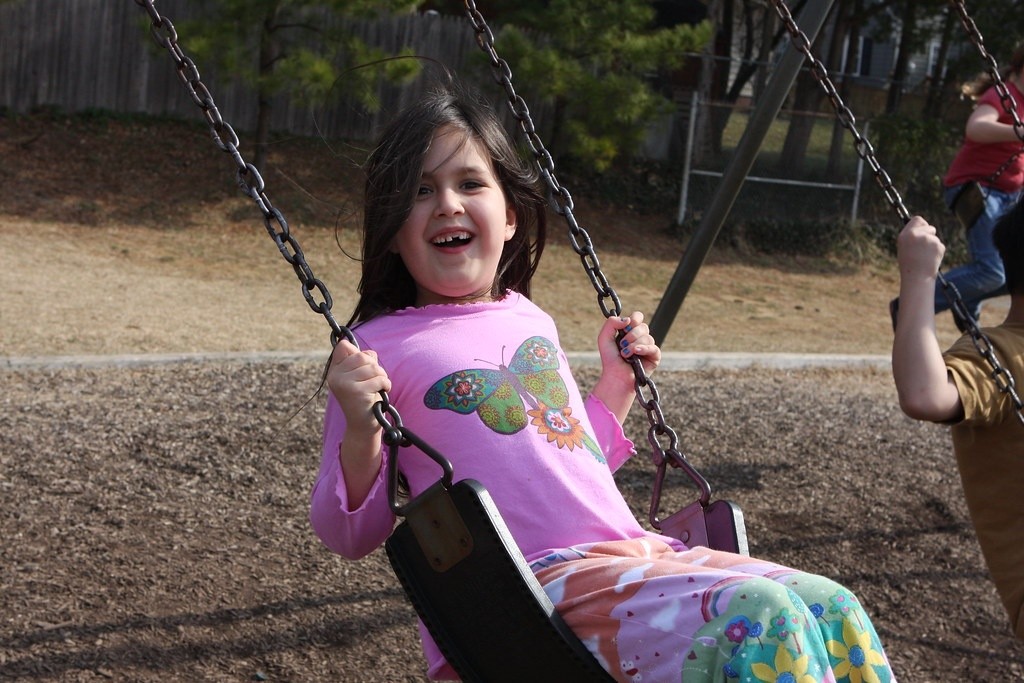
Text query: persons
308 92 894 683
889 39 1024 334
892 199 1024 644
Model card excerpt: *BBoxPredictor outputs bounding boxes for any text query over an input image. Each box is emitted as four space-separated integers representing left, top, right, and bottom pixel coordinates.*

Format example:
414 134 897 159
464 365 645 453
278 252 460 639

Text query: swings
950 146 1024 233
140 0 753 683
769 1 1024 425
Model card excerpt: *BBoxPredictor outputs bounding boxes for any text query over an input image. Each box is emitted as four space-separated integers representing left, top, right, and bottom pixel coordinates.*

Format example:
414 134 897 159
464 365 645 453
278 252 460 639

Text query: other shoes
890 297 900 334
